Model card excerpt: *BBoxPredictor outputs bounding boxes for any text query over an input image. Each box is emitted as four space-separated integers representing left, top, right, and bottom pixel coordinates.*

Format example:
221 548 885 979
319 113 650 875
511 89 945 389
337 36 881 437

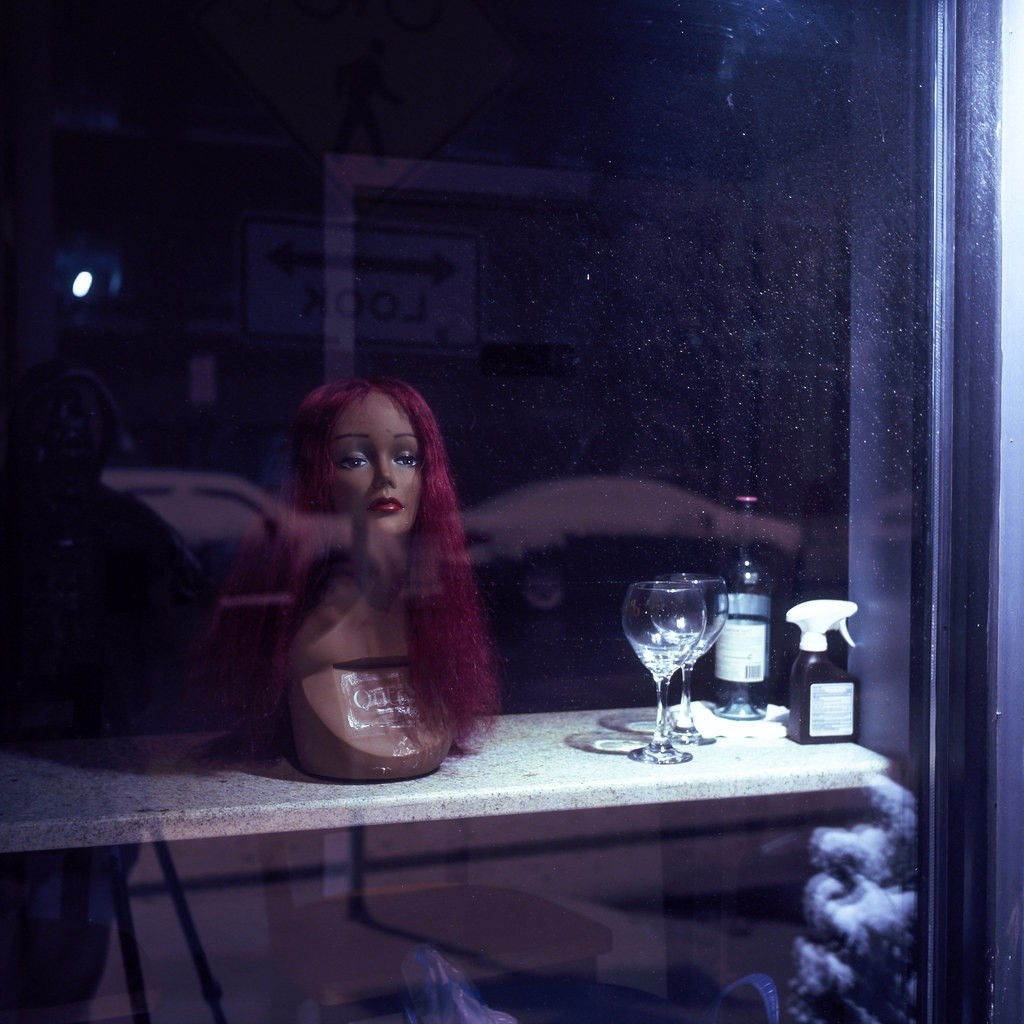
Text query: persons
134 376 503 785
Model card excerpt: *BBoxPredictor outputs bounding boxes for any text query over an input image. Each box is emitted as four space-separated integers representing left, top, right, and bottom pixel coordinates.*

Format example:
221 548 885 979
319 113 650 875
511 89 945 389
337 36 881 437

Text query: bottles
712 492 776 721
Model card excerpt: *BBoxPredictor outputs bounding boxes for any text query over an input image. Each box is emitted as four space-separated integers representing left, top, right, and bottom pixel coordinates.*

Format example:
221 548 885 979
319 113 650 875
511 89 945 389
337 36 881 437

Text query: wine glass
620 578 709 765
651 571 730 746
